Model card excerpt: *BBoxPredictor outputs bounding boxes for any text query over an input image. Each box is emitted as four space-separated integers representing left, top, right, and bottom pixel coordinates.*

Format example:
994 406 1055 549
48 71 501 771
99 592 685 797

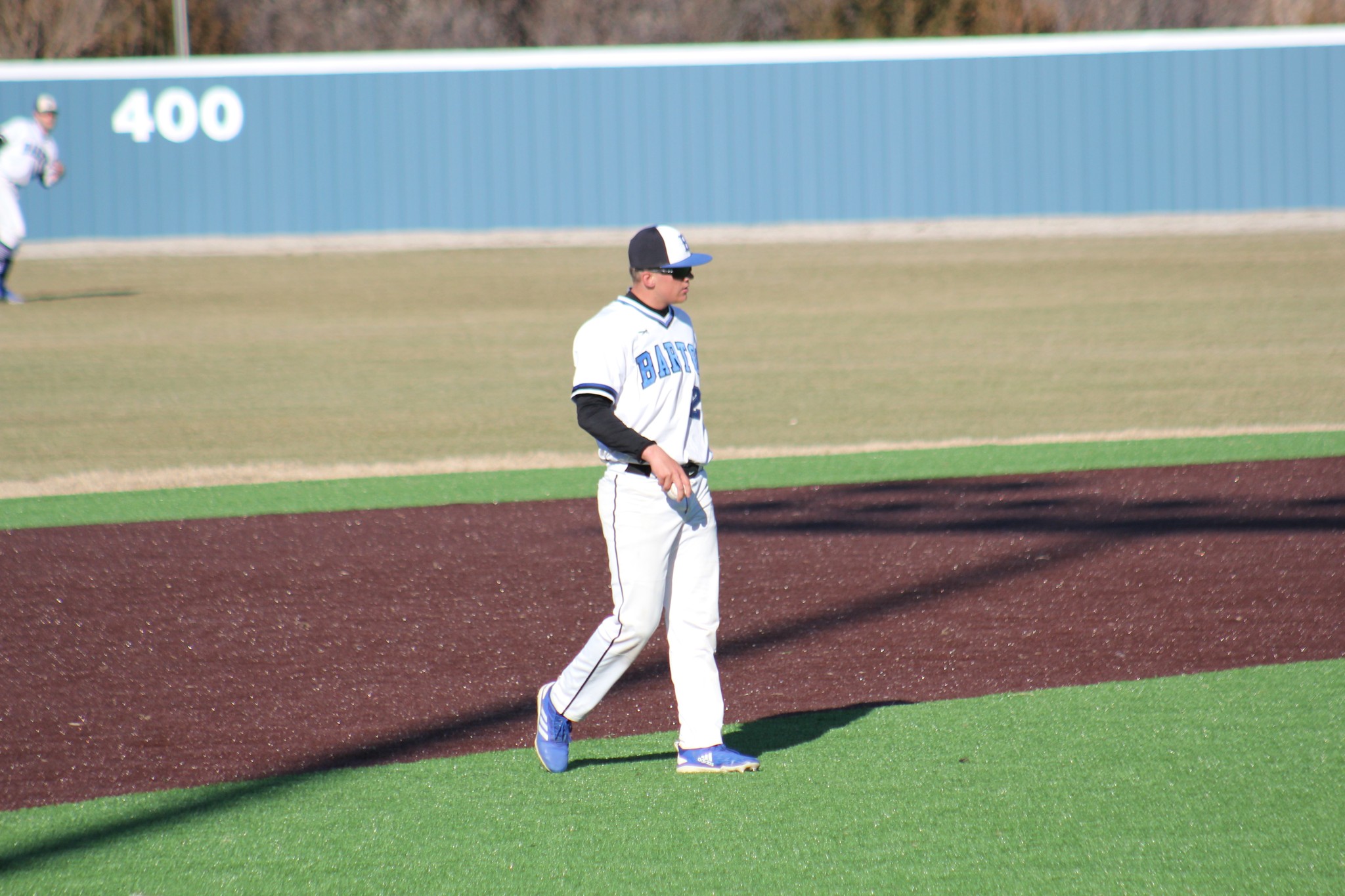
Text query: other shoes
0 291 25 305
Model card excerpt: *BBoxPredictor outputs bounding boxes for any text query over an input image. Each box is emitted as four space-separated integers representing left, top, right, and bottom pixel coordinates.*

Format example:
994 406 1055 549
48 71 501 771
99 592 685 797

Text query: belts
624 464 699 479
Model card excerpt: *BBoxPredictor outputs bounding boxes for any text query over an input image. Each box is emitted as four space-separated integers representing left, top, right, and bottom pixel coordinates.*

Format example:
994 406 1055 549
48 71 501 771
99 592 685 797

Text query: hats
629 225 712 268
35 94 58 111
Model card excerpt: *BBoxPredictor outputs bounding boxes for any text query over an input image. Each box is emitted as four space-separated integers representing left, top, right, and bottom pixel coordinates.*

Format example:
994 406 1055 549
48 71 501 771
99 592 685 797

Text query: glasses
636 267 692 280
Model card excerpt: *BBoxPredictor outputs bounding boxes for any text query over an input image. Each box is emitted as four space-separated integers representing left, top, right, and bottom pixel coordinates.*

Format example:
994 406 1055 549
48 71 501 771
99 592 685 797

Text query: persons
0 94 62 304
536 225 759 774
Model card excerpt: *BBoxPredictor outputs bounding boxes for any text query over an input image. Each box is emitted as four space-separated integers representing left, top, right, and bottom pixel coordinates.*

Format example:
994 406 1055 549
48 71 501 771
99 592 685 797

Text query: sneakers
674 742 760 773
534 682 572 774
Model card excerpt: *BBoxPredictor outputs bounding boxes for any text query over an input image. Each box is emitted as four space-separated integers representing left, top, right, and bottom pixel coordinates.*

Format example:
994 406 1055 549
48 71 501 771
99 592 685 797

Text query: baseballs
667 483 687 503
